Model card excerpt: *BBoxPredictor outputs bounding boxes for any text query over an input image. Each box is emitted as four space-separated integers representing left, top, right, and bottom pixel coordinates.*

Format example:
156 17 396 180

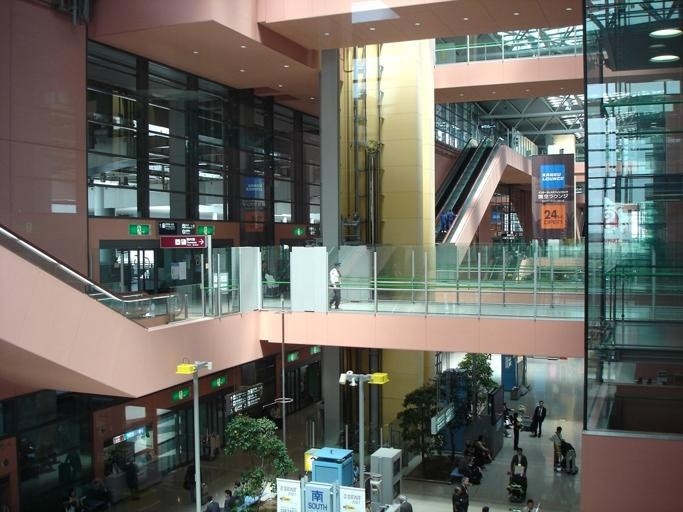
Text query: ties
538 407 541 418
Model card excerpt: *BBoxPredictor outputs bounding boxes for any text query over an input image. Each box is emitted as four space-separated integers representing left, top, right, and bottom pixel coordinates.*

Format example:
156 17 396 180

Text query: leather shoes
533 434 537 437
538 432 541 438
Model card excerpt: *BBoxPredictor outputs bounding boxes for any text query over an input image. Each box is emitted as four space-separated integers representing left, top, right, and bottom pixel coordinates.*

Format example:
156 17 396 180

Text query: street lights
174 359 214 512
336 370 390 495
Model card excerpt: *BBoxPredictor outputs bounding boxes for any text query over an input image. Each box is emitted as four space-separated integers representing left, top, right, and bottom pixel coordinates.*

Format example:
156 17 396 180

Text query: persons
440 211 448 233
559 438 577 473
549 426 564 467
329 262 342 309
20 430 141 512
447 210 458 230
398 412 542 511
530 401 546 438
271 405 281 425
261 258 290 298
183 456 245 512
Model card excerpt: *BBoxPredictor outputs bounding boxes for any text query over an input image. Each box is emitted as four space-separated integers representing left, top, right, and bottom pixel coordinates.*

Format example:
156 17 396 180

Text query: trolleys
506 474 527 504
518 404 533 432
557 442 578 476
